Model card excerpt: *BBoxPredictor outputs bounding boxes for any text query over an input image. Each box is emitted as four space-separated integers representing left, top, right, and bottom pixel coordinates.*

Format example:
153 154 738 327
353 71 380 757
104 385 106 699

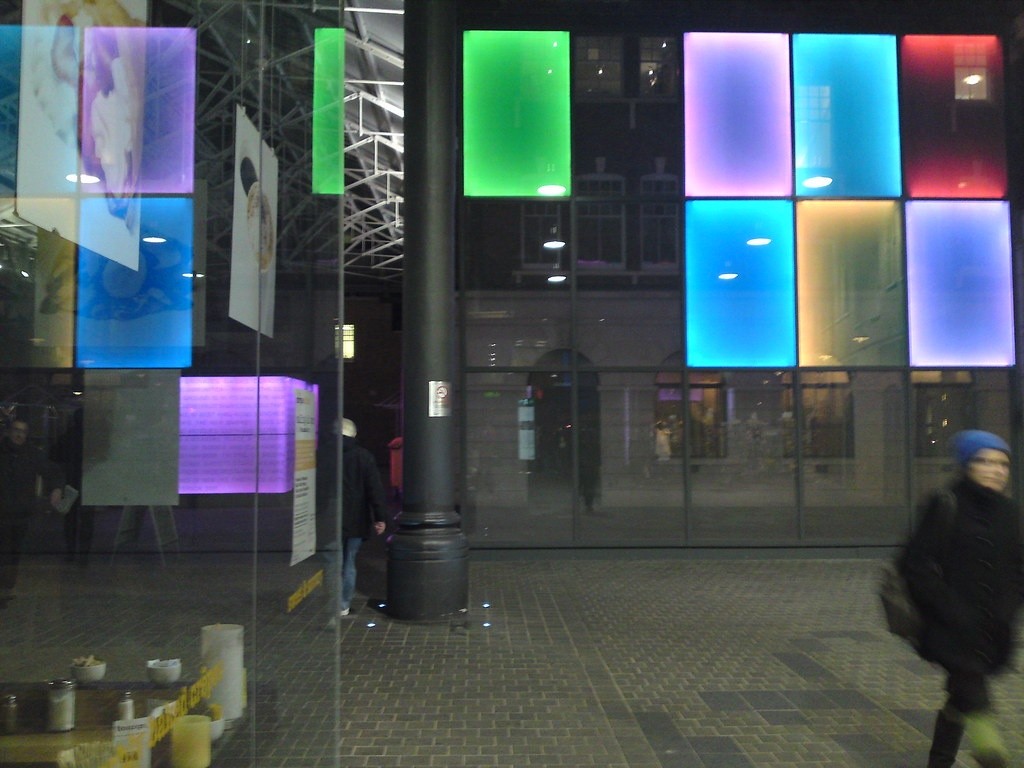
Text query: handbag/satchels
879 563 925 639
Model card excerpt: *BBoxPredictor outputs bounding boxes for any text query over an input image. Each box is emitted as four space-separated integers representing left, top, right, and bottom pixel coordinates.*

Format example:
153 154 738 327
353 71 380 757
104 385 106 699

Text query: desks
0 647 239 768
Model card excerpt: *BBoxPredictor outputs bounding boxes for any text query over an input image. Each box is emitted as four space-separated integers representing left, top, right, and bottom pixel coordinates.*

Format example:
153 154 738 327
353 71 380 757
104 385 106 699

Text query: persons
0 417 35 599
88 30 134 219
656 411 705 472
110 375 180 567
316 417 386 621
51 406 96 567
896 431 1024 768
578 425 602 510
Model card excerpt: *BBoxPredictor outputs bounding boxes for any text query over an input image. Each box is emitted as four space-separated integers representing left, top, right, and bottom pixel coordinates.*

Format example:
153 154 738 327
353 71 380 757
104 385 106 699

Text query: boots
963 715 1008 768
927 711 966 768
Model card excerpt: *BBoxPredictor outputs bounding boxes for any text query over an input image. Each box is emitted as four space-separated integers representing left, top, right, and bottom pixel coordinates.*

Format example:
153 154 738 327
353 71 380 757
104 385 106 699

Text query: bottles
44 674 76 732
0 693 18 735
171 714 212 768
116 690 136 720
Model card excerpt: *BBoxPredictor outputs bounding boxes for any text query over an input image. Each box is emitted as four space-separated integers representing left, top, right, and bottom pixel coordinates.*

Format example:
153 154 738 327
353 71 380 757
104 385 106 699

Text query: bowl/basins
69 660 107 684
202 718 225 744
147 661 182 688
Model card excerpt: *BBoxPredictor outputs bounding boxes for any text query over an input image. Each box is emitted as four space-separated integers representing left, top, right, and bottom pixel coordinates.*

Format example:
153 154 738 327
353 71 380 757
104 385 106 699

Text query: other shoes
339 608 351 616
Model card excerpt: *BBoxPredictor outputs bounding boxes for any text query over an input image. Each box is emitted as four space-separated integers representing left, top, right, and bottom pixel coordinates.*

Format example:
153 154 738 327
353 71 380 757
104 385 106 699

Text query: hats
331 418 357 438
953 429 1012 474
